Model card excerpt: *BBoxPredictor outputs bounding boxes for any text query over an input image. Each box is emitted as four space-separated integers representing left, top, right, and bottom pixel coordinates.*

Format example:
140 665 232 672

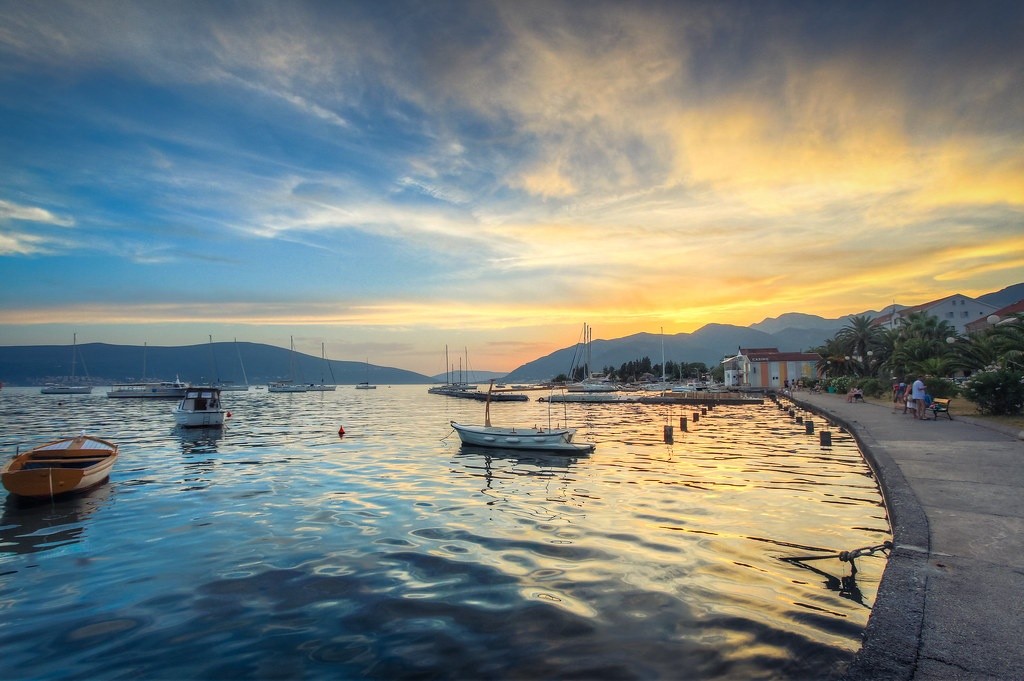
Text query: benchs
815 386 821 394
925 398 953 421
856 393 864 403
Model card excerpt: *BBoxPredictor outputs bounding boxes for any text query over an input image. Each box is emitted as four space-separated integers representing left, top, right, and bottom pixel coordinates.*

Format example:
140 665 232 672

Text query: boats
449 418 596 451
356 356 376 390
190 335 249 391
38 332 93 394
311 339 337 391
267 335 307 393
107 341 190 397
170 385 225 427
0 430 120 497
568 321 707 392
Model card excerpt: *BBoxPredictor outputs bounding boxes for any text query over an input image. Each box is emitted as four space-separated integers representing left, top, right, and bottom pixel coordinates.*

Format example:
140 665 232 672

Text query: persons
808 382 819 395
782 378 795 391
892 375 931 420
846 385 864 403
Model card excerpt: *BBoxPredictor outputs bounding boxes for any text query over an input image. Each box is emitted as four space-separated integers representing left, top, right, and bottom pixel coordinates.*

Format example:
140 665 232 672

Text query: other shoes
892 411 895 414
848 401 851 403
918 417 921 420
903 411 906 414
923 417 928 420
912 416 917 418
